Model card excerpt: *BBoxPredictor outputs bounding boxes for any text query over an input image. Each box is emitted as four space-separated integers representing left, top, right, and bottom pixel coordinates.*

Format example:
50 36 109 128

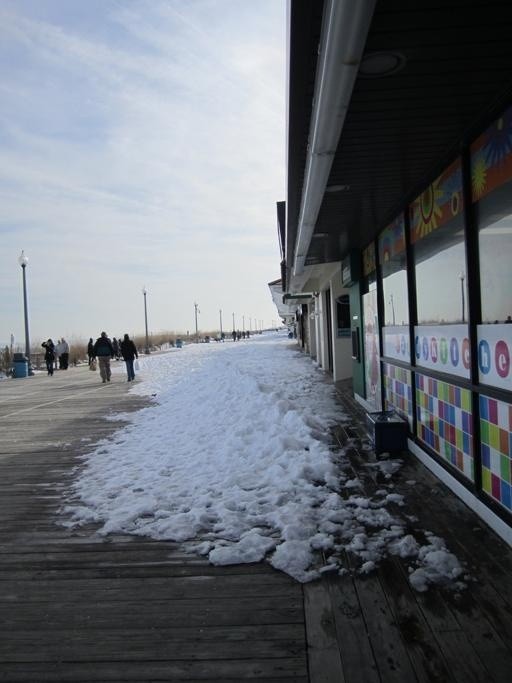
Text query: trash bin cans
12 353 29 378
176 339 183 348
204 336 211 343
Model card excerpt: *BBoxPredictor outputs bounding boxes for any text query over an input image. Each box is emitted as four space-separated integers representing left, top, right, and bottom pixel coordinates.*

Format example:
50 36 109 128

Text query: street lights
391 293 396 325
458 271 465 324
18 249 36 377
219 309 222 340
242 313 245 335
142 285 152 354
231 311 235 338
194 302 198 344
248 317 264 334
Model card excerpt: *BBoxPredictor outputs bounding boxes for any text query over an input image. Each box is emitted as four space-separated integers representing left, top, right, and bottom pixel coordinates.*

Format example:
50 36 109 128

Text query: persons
41 338 55 376
107 335 125 360
87 337 96 366
94 331 115 383
60 337 70 369
215 329 249 343
56 339 63 368
120 333 139 381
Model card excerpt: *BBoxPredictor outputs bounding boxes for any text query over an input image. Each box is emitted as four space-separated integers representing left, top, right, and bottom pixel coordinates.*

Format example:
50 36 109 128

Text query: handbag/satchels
90 358 97 370
135 359 141 370
